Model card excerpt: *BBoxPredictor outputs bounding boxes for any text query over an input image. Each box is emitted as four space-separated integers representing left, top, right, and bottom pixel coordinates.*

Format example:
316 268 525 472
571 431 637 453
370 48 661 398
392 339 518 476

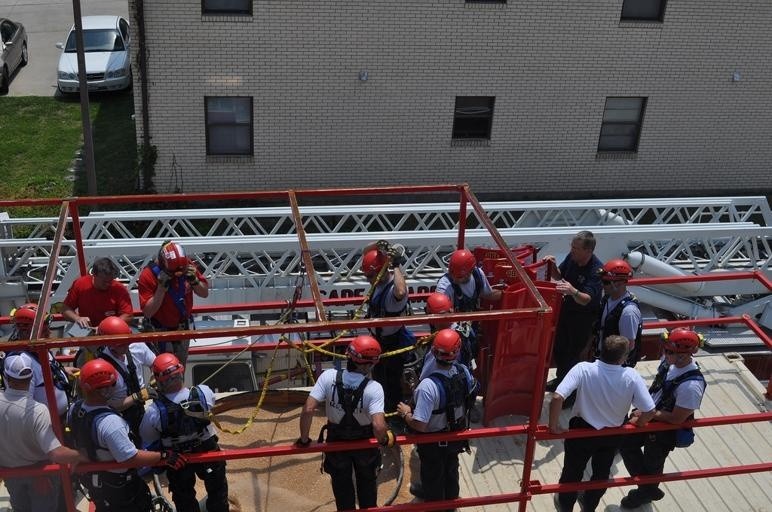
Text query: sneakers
467 405 480 423
621 486 665 508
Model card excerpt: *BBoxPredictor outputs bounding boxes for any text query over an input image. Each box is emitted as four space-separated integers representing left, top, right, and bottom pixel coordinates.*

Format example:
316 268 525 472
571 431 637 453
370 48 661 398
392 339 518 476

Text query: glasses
601 280 613 285
664 349 674 355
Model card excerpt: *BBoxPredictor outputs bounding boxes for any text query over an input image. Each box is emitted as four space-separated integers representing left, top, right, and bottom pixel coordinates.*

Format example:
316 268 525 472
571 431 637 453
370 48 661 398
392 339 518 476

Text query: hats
4 351 34 380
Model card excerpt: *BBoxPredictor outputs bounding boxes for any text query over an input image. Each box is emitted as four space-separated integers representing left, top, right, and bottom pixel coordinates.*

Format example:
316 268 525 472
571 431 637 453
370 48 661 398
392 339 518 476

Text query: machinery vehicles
0 191 771 397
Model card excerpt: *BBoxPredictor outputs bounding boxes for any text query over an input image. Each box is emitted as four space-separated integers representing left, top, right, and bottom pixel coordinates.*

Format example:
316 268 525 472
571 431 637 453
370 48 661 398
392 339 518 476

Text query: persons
616 324 709 510
139 353 229 512
0 304 91 510
433 248 506 426
394 327 487 512
289 333 398 510
410 289 479 384
539 228 606 410
134 239 209 365
547 332 659 511
359 239 410 414
578 256 644 369
66 359 186 510
56 258 158 449
571 288 580 302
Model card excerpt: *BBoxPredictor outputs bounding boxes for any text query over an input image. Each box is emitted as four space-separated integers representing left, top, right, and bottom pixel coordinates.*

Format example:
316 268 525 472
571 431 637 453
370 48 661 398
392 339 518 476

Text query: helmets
361 250 389 277
159 240 187 278
662 326 699 353
449 248 473 282
79 316 184 400
14 303 53 330
426 291 462 361
345 336 382 364
597 259 633 279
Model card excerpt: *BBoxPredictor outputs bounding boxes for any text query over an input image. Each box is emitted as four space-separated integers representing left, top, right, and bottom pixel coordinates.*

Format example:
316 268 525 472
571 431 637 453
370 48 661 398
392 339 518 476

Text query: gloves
156 271 174 292
185 264 199 286
294 436 312 446
376 239 406 267
380 430 396 449
162 448 188 471
132 389 159 403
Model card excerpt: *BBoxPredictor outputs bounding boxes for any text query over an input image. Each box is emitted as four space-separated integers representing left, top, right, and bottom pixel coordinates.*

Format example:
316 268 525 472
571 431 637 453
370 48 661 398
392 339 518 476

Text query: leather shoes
410 483 426 498
545 378 562 392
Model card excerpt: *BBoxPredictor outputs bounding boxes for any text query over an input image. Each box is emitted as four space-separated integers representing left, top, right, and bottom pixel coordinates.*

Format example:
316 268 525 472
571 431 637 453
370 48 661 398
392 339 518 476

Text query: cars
55 15 131 95
0 17 29 95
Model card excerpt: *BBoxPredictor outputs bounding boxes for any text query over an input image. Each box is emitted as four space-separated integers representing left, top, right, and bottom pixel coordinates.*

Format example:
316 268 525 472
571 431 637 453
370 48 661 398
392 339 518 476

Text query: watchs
400 409 412 420
